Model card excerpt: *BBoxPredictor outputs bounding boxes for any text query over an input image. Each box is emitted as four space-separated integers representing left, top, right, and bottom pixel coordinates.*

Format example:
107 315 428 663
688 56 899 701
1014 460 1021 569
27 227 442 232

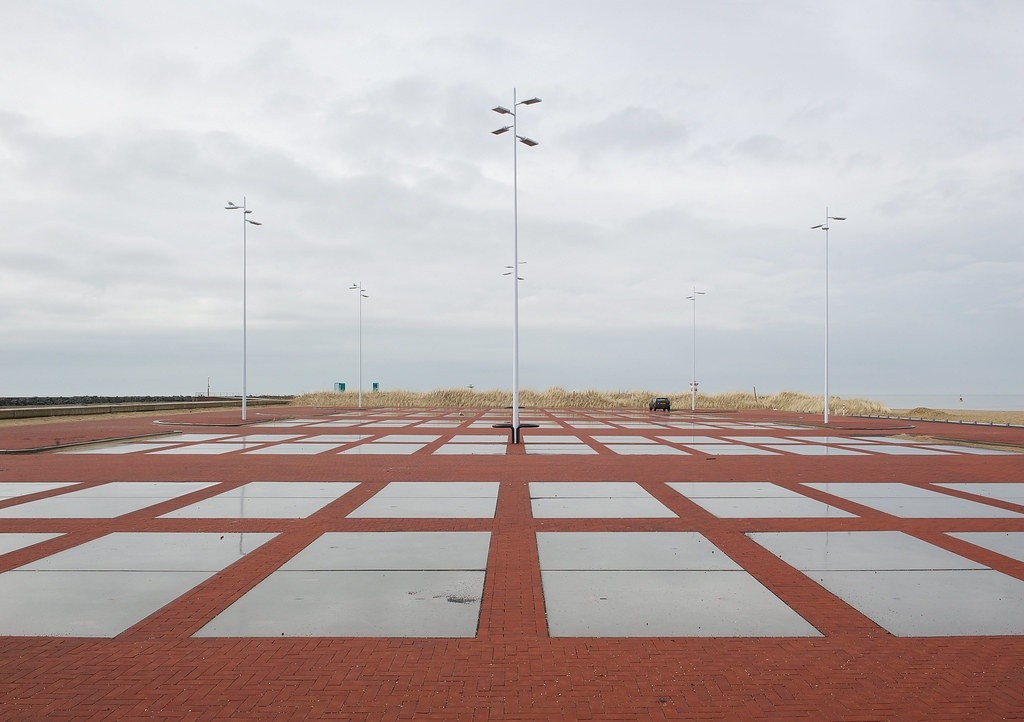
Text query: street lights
686 285 705 410
225 196 262 421
492 86 542 445
811 207 846 423
349 280 369 409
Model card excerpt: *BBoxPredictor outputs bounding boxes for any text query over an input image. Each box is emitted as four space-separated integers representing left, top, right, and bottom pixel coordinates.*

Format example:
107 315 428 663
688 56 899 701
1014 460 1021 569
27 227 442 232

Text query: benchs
492 423 539 444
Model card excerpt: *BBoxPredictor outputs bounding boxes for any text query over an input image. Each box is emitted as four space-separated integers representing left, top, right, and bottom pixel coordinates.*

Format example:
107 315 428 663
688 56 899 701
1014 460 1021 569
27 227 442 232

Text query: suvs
648 396 670 411
